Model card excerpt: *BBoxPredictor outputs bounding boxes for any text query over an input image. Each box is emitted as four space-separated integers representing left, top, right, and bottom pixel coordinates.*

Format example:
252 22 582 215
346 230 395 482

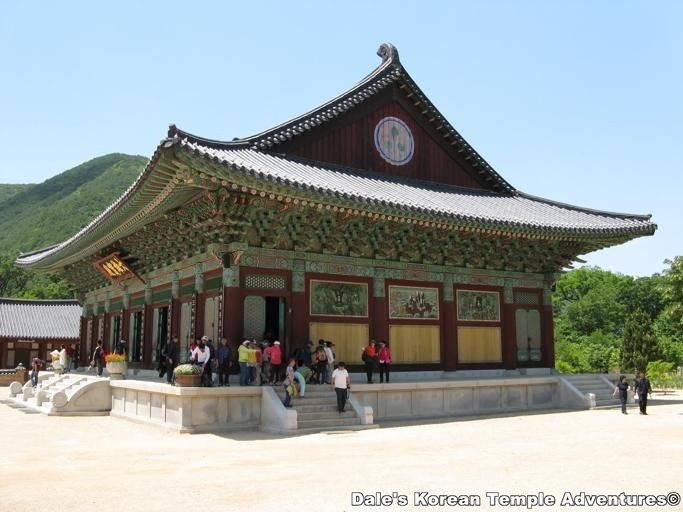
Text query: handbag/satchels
222 358 241 375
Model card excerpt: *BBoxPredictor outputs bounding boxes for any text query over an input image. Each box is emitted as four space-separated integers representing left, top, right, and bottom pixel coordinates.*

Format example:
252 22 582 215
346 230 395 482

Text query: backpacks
294 346 304 363
362 348 367 361
98 347 106 360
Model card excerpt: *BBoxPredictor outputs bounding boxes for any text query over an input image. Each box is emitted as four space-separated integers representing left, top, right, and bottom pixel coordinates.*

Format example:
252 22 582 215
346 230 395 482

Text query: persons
612 375 630 414
30 356 42 386
88 339 104 375
142 329 350 413
65 344 75 372
56 343 67 374
72 350 79 369
376 341 391 383
634 372 652 415
112 337 128 355
361 339 377 383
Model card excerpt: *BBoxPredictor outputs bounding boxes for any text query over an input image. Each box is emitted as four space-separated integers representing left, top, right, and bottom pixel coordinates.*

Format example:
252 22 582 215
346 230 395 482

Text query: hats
201 336 209 341
